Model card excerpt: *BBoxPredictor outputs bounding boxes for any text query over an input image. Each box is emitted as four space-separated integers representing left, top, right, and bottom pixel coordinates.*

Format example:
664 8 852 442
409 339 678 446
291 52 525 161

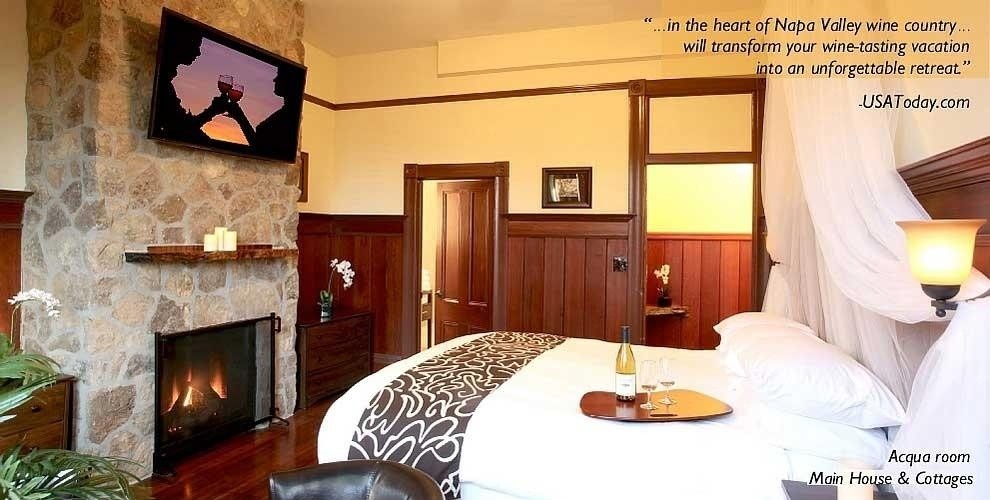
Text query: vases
657 295 672 308
318 290 333 322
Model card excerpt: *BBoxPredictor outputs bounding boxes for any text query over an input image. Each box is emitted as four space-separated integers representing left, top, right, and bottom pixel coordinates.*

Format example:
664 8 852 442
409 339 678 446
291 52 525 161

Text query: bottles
615 325 638 402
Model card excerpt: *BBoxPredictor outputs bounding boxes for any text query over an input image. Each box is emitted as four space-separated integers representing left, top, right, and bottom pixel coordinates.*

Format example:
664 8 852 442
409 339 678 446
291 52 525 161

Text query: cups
659 355 678 405
639 358 657 410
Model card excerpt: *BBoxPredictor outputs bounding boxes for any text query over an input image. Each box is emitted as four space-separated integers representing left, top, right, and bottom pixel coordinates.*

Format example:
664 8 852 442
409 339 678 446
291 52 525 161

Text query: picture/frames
299 151 309 202
541 166 592 209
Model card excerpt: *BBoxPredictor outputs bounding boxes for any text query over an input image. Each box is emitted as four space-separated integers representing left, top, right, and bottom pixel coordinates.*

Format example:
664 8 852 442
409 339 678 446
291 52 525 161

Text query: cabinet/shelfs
125 249 299 263
296 309 372 410
1 373 74 456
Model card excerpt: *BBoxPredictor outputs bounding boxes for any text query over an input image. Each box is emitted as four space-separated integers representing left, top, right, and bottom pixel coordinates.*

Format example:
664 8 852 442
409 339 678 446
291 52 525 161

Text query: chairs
269 461 443 500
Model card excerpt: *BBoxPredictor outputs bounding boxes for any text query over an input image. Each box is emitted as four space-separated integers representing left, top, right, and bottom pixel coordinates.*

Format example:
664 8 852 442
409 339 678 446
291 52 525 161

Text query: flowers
1 289 62 344
327 257 355 293
654 264 672 296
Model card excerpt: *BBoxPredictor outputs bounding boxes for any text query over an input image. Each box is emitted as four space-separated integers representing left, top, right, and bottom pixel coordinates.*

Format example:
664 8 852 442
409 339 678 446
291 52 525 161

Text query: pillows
714 312 907 430
716 345 888 470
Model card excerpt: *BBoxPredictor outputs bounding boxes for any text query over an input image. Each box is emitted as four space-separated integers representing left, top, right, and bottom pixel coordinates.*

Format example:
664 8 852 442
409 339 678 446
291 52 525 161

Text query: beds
317 332 903 499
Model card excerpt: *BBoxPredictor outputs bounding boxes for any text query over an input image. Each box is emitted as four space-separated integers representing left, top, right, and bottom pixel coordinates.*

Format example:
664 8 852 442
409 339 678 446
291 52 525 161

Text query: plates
579 389 733 424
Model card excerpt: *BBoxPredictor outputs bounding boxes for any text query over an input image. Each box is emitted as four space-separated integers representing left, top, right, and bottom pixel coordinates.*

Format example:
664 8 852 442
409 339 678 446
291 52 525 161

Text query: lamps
895 219 990 317
